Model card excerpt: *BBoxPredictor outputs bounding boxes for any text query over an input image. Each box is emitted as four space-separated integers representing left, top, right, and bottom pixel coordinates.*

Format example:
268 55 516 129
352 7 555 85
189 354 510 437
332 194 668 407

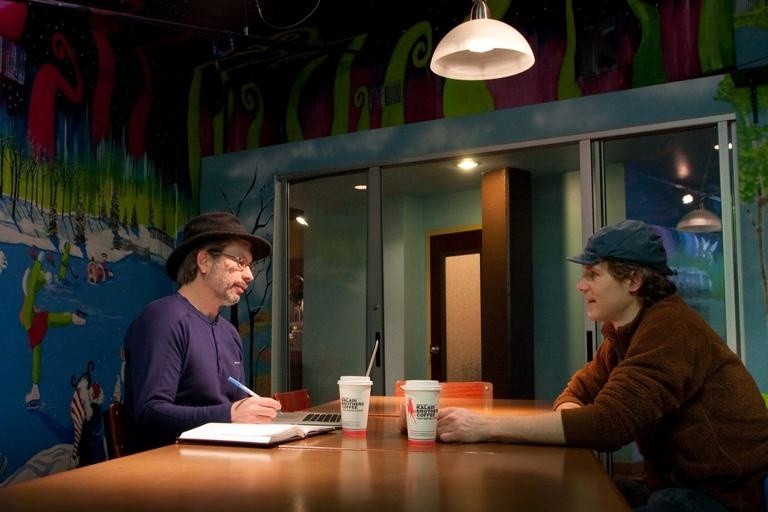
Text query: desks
0 396 628 512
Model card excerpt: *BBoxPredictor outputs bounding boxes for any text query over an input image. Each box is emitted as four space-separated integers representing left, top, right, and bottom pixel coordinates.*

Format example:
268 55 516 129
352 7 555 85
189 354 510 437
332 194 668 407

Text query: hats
565 220 674 276
166 212 273 281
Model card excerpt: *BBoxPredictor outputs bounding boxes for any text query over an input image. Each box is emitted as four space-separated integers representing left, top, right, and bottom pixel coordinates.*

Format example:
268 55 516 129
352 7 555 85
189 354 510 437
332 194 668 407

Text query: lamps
430 1 536 81
676 200 722 232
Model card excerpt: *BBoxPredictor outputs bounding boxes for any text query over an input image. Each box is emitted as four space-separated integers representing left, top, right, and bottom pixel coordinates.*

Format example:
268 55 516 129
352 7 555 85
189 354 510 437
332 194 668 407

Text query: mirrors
593 113 737 363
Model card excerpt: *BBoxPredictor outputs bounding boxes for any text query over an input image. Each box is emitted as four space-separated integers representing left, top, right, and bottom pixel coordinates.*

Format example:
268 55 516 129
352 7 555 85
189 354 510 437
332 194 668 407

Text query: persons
120 210 282 453
288 274 304 323
438 217 767 512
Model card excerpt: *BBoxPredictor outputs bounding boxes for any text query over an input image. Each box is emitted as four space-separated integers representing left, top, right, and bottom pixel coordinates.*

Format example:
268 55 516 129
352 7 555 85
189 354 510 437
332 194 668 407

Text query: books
177 420 336 447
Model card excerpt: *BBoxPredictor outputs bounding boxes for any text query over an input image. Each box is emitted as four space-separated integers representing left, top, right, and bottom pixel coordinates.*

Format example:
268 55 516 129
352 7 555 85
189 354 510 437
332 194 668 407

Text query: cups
400 379 441 444
337 375 372 435
403 447 445 511
337 435 375 505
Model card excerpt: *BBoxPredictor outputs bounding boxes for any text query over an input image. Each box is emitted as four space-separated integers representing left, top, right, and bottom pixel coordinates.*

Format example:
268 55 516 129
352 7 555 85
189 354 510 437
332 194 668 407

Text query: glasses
210 252 253 270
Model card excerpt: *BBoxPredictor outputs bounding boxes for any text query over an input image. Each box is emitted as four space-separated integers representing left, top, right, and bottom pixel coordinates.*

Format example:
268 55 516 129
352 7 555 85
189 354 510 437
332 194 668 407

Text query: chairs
394 380 494 400
274 389 312 412
110 401 124 456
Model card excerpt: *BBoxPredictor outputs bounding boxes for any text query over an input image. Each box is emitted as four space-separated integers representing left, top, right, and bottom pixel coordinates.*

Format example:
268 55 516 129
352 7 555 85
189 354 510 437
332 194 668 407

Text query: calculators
298 411 343 427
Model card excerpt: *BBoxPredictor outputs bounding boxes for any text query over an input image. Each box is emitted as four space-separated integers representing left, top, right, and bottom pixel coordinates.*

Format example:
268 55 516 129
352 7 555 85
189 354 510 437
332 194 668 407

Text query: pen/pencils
227 377 284 418
